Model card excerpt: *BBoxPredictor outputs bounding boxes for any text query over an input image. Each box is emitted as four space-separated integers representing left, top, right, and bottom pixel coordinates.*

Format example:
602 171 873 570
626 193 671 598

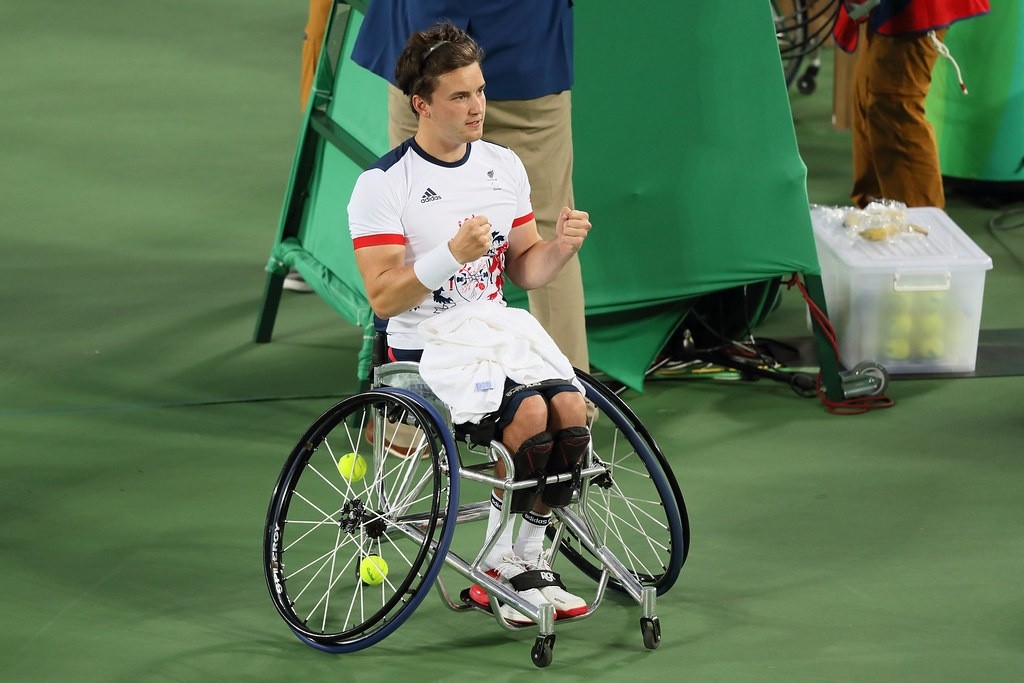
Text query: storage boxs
810 208 993 373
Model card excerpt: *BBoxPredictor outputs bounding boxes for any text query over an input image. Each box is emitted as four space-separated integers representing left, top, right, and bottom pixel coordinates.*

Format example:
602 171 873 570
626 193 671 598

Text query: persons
351 1 602 427
340 18 592 626
841 0 993 238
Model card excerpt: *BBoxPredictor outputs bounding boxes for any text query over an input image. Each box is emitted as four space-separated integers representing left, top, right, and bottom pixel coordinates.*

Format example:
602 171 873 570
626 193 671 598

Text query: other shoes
365 419 430 460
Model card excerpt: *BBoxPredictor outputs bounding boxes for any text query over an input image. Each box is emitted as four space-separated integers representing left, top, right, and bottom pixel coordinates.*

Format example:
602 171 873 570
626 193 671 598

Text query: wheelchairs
263 310 692 667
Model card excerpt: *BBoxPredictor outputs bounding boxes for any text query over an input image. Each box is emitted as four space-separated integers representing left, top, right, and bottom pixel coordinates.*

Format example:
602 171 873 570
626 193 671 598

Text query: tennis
884 272 951 368
337 452 367 481
359 554 387 586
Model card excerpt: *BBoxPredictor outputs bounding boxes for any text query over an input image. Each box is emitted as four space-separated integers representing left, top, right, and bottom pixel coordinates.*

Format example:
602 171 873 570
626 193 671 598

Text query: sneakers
469 548 589 624
